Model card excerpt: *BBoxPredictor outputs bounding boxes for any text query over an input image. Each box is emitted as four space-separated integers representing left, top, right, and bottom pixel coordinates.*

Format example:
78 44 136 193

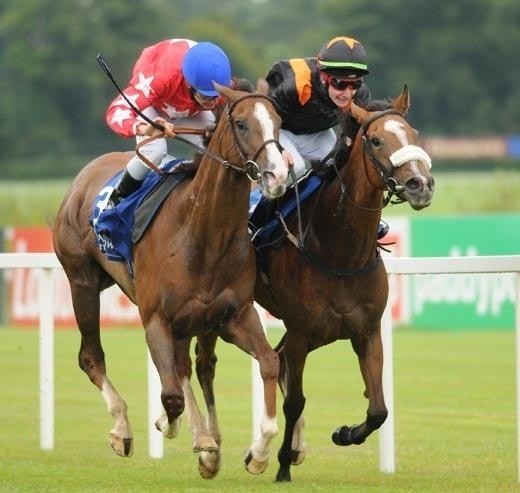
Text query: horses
52 78 290 481
194 82 435 482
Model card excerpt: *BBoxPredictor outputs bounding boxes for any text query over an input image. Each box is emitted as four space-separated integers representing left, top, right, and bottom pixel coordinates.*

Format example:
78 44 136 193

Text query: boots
94 167 145 245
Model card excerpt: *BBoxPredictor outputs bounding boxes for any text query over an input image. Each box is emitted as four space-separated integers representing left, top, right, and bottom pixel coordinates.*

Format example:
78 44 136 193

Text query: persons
247 36 389 238
98 39 232 238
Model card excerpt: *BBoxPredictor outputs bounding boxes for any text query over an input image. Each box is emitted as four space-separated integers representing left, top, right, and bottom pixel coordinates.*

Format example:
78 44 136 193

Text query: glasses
329 77 362 90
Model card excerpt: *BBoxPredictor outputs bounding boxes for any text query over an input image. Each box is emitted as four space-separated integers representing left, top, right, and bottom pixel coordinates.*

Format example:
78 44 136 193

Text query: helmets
183 43 232 97
317 36 370 79
